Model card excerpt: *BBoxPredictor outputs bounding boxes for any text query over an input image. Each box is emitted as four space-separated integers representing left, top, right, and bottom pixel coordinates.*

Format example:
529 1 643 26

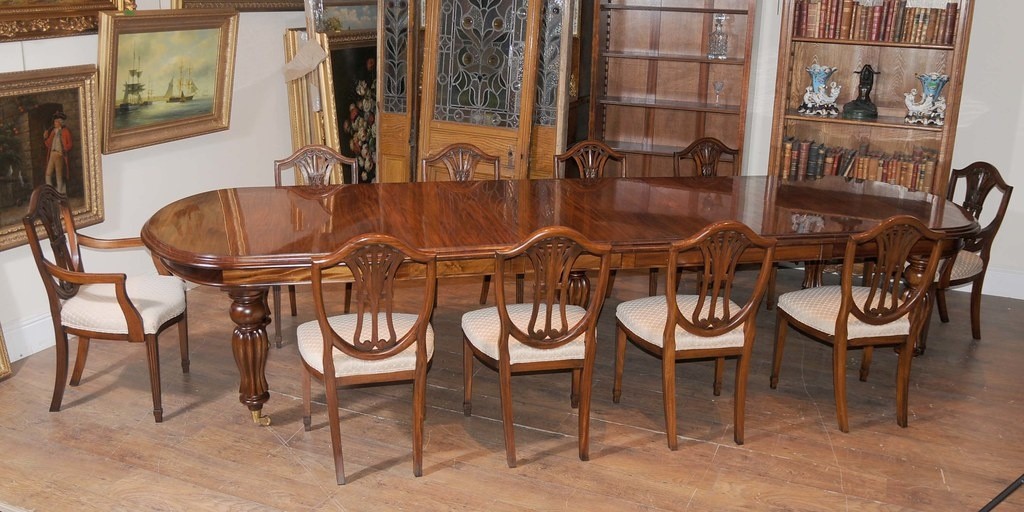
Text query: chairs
22 184 189 426
648 136 740 298
274 144 358 348
614 220 776 455
420 141 501 307
901 160 1015 344
552 140 629 299
771 216 947 436
458 226 612 472
294 230 440 488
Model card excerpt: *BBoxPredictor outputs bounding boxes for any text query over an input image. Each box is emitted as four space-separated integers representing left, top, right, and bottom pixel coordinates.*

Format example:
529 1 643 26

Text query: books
779 136 938 192
793 0 960 45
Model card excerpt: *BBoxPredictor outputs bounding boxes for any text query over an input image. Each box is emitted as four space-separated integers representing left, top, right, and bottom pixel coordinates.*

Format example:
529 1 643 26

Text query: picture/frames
0 64 105 254
279 0 425 187
170 0 306 13
149 187 251 256
98 9 242 156
0 0 136 43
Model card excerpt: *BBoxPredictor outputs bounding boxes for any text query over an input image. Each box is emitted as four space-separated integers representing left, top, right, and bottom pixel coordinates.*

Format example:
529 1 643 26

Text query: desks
139 172 983 429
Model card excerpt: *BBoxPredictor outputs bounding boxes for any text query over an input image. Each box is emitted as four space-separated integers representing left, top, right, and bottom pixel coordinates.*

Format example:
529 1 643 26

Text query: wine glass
712 81 724 108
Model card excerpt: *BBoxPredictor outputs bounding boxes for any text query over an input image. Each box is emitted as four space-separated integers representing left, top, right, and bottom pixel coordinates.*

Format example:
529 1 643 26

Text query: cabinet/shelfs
587 0 759 178
600 180 746 241
769 0 976 274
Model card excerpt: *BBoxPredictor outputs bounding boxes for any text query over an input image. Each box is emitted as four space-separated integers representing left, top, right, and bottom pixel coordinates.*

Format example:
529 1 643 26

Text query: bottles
707 12 728 60
